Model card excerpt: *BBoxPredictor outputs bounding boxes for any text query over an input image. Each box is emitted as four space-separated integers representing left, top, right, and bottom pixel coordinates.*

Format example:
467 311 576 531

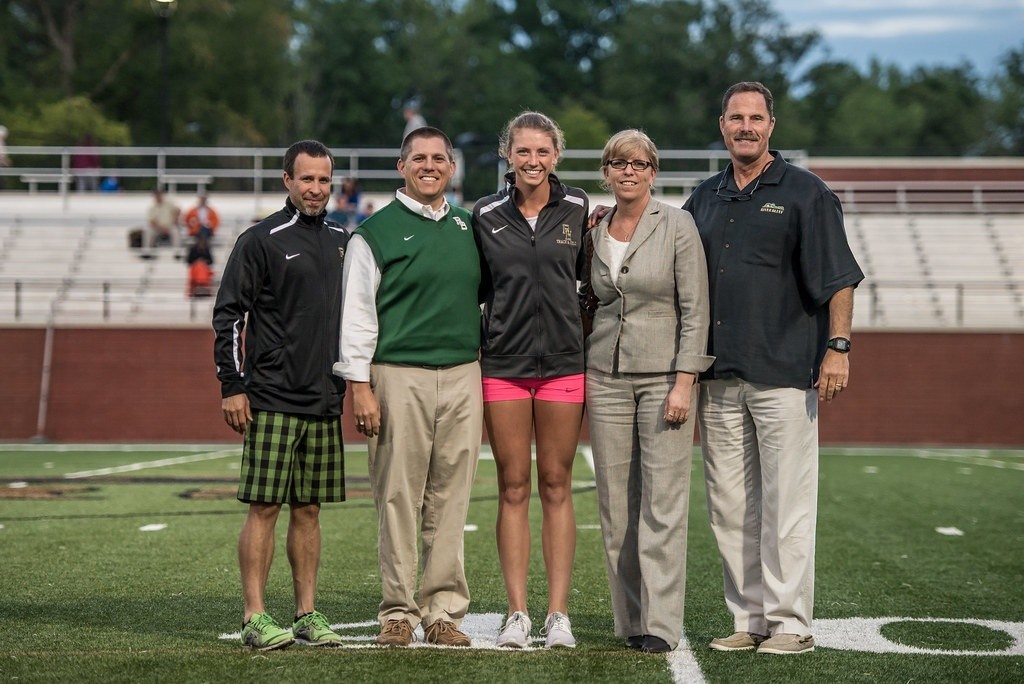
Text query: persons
331 125 484 647
342 176 360 206
186 235 213 267
472 110 606 646
183 192 218 245
209 141 352 647
355 202 375 222
400 99 428 149
579 129 717 655
328 195 354 228
100 176 123 192
186 255 214 298
135 188 183 258
71 133 103 190
585 80 868 654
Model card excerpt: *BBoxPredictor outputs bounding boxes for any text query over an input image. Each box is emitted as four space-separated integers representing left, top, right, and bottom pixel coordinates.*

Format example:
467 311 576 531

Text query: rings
360 422 364 426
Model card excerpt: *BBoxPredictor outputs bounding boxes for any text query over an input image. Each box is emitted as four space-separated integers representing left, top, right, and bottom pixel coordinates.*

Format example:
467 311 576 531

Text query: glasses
716 160 774 202
606 159 653 170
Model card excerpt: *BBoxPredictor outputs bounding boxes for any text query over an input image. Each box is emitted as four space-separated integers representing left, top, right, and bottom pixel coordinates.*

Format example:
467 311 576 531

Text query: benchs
838 216 1023 325
0 193 294 324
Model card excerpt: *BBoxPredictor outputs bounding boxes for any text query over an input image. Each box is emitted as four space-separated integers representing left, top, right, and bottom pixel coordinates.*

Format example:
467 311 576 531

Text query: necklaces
616 214 637 241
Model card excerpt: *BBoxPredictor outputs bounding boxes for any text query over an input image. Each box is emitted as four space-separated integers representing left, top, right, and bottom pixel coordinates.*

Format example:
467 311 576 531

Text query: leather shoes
625 635 642 648
642 635 677 653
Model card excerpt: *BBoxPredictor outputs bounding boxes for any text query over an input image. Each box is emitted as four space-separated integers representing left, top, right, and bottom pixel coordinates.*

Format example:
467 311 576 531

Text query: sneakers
424 619 471 645
240 612 296 650
292 610 344 647
377 620 413 645
498 610 533 648
757 634 815 654
544 612 576 648
709 632 770 651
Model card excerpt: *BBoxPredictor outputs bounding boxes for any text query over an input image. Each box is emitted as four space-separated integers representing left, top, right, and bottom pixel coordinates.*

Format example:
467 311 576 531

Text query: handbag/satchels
578 284 597 340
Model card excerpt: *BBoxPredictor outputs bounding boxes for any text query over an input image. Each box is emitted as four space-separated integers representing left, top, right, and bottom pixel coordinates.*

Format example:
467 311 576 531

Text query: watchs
827 336 852 353
